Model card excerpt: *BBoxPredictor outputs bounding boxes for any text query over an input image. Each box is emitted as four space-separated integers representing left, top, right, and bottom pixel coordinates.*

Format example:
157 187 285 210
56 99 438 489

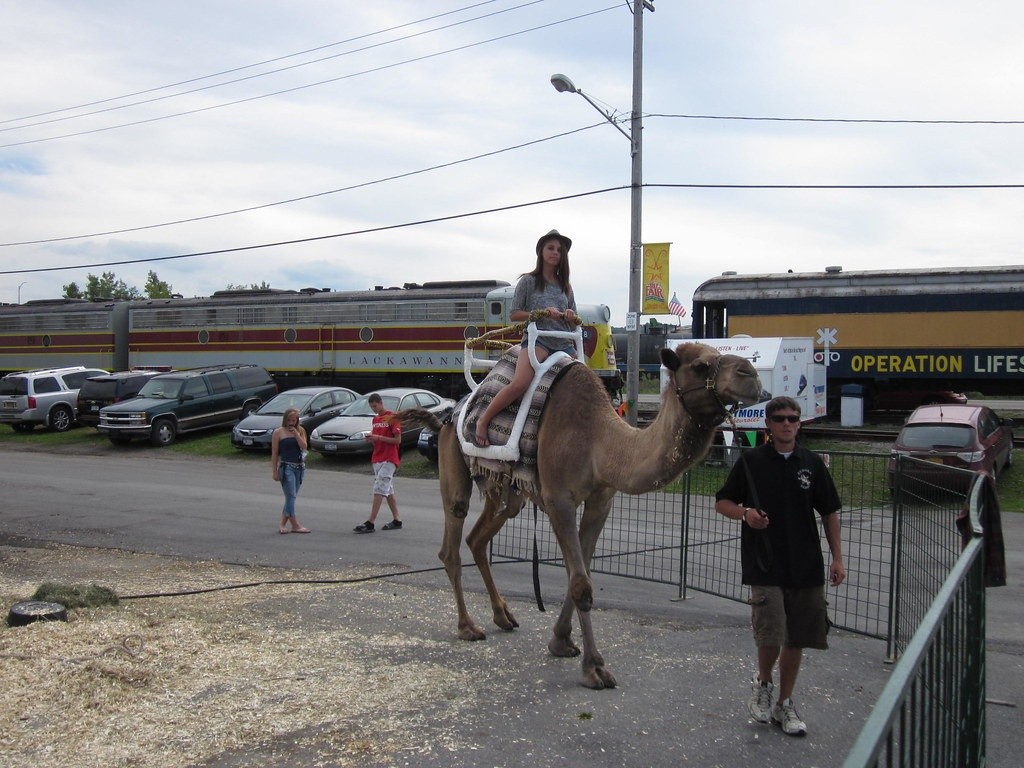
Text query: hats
536 229 572 255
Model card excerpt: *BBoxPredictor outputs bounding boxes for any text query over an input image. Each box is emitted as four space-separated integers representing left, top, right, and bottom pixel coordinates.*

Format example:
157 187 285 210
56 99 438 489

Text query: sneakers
771 697 808 736
748 672 774 723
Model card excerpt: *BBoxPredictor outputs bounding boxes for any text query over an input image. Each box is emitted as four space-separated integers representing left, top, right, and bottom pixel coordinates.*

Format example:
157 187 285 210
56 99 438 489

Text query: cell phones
365 436 370 437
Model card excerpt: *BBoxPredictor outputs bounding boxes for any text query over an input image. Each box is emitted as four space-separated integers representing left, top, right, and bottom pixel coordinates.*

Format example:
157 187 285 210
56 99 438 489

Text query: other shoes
279 526 289 534
291 525 310 533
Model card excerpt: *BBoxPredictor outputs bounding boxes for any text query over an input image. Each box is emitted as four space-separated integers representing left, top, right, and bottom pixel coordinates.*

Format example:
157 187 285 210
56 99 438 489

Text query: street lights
552 72 643 428
17 281 28 304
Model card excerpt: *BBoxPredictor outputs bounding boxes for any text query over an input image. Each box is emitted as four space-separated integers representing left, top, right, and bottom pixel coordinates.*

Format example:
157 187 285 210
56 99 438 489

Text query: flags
667 295 686 317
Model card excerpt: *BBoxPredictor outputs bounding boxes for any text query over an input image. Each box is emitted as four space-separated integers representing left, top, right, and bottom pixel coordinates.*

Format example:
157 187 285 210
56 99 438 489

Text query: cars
418 405 452 463
888 403 1015 507
309 387 456 464
231 385 364 455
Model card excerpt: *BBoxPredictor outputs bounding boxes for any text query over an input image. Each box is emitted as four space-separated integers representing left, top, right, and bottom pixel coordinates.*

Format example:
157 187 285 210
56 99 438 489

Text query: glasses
768 414 801 423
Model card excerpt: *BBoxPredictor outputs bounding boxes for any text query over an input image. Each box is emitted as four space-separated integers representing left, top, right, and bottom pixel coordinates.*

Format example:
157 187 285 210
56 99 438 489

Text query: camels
383 342 761 690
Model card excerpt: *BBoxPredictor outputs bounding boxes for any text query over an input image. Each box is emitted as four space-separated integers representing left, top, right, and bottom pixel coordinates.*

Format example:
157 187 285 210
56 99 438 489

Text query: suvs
75 365 174 432
0 364 111 434
94 363 278 446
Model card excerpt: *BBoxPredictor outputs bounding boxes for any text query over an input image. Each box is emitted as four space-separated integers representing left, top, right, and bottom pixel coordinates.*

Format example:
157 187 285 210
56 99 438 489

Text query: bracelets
743 508 751 520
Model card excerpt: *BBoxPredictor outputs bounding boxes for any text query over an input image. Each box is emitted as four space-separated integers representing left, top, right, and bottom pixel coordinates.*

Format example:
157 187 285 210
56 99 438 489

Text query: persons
271 409 311 533
713 396 846 735
353 394 402 533
468 230 579 448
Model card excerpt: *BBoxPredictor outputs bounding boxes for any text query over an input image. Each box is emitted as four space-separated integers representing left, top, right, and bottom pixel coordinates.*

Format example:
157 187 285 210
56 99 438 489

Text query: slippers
467 423 489 448
353 523 375 533
381 522 402 530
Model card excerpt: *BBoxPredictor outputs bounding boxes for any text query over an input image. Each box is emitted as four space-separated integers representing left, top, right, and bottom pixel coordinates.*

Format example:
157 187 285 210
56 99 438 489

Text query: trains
0 263 1024 414
